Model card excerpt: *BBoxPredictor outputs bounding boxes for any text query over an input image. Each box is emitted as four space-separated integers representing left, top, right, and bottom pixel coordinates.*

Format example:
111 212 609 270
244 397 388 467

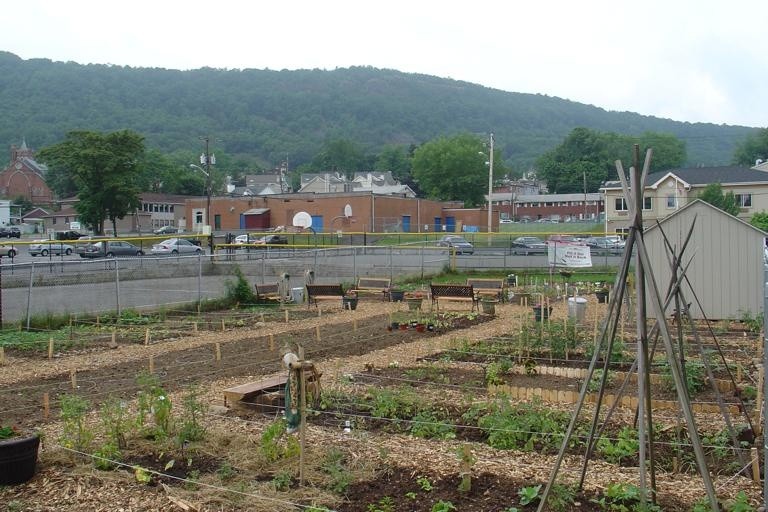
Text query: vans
75 236 120 257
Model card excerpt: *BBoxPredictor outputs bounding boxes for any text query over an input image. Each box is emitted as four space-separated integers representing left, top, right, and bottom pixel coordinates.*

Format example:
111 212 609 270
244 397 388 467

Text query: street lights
189 163 210 235
478 150 494 247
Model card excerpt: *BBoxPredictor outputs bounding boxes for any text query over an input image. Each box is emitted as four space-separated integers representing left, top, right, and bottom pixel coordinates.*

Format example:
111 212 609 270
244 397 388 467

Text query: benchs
351 277 390 303
305 284 353 310
465 278 504 304
429 284 480 313
253 283 279 306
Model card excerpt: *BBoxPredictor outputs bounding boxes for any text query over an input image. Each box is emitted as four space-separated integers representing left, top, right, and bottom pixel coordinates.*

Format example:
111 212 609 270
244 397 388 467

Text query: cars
153 226 178 235
499 214 597 224
0 244 19 258
187 239 201 247
253 235 288 249
0 227 21 239
511 232 627 260
53 230 88 241
84 241 146 259
231 235 258 249
151 237 202 255
28 239 74 257
439 235 474 255
